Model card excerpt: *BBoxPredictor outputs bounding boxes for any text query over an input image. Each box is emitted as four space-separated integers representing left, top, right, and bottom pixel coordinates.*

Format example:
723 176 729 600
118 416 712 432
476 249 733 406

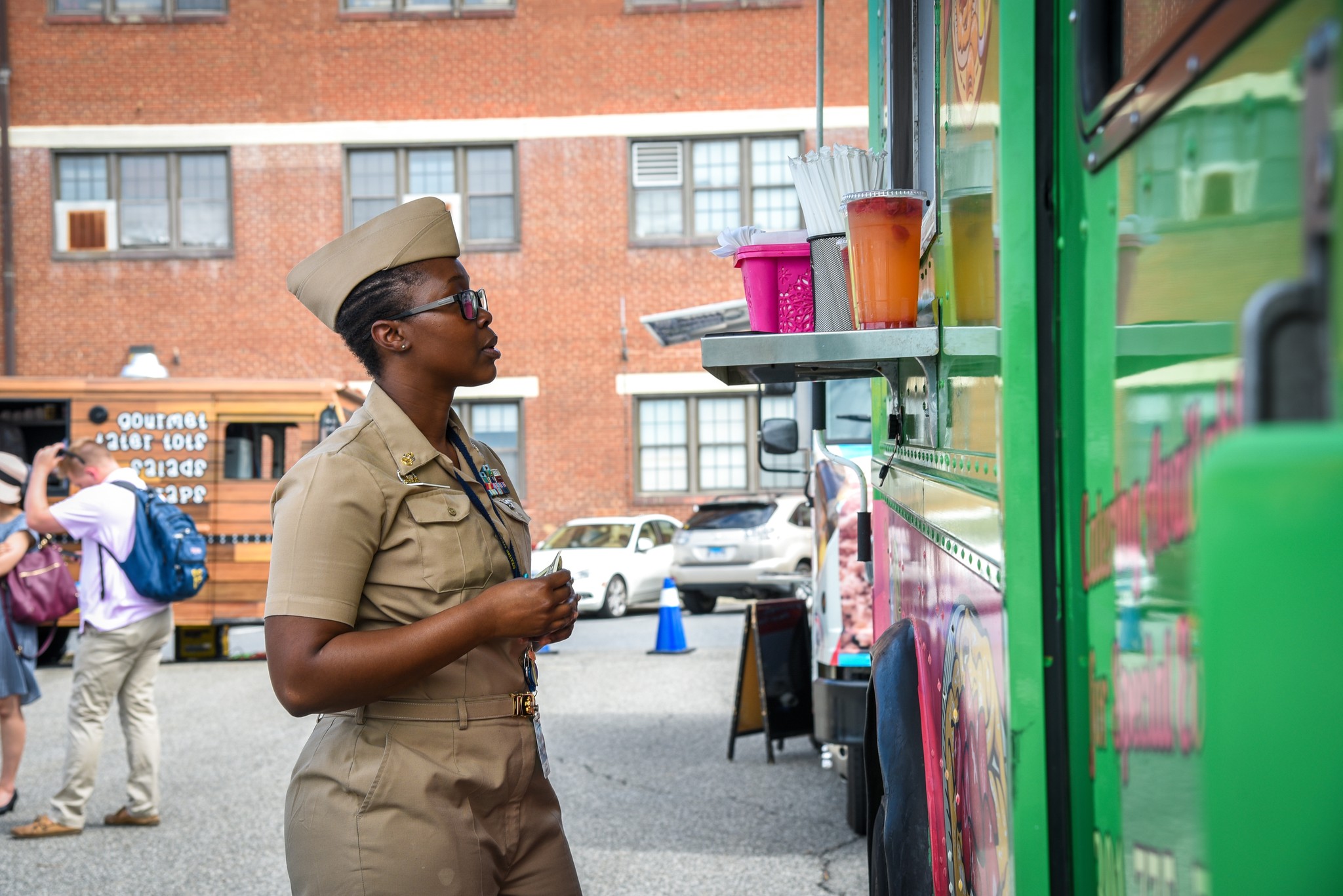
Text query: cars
526 513 684 620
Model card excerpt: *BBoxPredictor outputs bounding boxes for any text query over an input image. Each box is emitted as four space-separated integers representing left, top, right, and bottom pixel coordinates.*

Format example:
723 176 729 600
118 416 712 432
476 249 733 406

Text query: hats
0 451 28 504
286 197 460 334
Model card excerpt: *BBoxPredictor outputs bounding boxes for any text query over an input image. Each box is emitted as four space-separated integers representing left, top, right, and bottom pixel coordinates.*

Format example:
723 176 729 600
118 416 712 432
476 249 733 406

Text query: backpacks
93 480 209 603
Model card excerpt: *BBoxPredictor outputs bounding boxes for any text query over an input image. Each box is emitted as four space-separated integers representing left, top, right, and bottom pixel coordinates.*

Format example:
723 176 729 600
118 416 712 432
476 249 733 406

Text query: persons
0 448 80 813
258 196 581 896
12 439 208 838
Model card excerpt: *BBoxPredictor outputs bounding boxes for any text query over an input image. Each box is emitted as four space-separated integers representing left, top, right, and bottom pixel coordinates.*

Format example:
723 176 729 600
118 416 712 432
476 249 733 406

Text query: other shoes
11 811 83 838
103 807 161 825
0 787 19 815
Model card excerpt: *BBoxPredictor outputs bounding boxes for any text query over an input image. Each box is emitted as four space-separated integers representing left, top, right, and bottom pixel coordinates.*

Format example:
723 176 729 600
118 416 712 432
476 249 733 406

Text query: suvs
671 490 815 611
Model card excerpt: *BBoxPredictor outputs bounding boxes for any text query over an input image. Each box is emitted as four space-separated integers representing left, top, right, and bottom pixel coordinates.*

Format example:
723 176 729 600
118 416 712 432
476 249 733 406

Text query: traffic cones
644 578 698 657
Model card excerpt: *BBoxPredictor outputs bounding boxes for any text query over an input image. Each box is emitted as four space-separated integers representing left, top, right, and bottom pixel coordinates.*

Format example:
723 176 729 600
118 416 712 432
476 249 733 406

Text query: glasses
57 445 88 465
380 288 489 322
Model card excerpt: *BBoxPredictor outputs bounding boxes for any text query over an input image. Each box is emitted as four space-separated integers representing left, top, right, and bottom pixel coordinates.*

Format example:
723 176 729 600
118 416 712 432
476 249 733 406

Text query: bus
0 376 370 668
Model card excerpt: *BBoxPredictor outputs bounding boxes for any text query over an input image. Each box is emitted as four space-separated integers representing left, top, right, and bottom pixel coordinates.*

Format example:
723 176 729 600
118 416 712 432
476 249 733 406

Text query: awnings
644 301 745 347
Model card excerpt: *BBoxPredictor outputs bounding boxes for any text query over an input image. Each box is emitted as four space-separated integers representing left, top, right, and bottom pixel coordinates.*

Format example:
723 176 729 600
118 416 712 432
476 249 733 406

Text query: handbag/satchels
4 516 79 625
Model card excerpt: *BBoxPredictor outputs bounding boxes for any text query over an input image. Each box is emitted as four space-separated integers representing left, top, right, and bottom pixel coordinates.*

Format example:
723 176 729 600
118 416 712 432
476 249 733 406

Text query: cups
842 187 929 327
940 185 1000 327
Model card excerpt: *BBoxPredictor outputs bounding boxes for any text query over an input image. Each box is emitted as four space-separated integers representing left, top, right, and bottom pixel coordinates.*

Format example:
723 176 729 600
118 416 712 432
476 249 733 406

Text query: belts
331 691 537 721
